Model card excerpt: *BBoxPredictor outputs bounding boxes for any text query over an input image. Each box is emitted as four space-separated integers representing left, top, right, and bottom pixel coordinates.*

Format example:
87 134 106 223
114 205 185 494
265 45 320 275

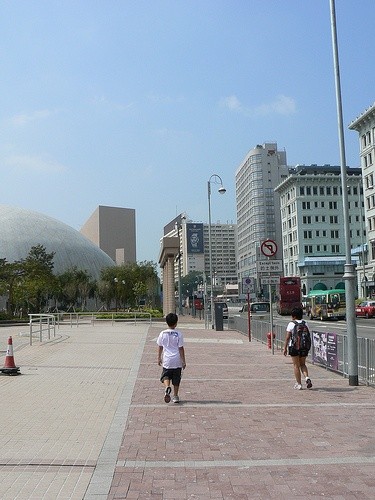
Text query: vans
239 302 273 323
210 302 228 319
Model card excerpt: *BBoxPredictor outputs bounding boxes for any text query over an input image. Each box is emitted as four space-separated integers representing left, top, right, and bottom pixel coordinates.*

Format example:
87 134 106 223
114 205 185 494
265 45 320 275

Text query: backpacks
291 320 311 351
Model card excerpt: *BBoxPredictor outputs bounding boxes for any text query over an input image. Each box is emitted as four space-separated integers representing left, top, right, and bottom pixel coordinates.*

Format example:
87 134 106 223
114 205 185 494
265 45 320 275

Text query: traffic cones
3 336 16 369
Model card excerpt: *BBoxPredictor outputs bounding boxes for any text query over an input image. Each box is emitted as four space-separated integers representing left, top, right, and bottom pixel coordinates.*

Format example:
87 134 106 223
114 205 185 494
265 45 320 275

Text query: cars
355 301 375 318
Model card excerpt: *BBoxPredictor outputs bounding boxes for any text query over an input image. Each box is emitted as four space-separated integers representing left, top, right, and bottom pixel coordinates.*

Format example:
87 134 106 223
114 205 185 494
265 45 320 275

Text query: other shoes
305 377 312 389
294 384 302 389
173 397 179 403
164 387 171 403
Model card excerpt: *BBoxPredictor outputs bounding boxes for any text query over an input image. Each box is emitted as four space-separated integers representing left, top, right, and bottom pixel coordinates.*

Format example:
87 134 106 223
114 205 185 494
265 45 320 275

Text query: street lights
114 278 119 315
208 174 226 329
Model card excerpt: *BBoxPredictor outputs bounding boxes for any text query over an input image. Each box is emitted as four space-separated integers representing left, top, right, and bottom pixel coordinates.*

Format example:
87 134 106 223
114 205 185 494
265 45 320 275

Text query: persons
155 312 186 403
282 306 314 391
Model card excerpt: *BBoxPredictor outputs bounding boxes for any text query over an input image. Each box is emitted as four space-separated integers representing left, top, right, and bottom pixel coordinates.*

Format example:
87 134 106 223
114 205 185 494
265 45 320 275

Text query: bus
306 289 346 321
275 276 303 315
